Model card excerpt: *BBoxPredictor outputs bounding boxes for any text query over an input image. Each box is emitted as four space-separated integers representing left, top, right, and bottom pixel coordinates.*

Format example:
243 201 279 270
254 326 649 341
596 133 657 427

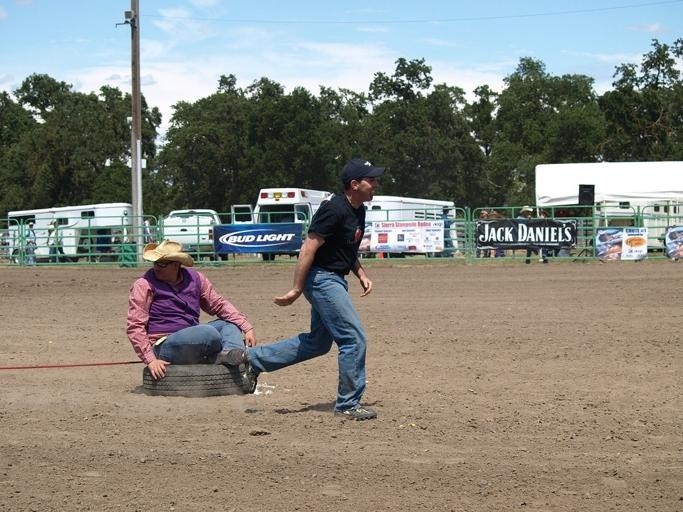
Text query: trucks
231 186 336 259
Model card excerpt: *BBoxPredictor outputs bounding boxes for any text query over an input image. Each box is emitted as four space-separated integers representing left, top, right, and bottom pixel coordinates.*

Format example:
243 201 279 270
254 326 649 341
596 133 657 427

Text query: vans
160 208 228 261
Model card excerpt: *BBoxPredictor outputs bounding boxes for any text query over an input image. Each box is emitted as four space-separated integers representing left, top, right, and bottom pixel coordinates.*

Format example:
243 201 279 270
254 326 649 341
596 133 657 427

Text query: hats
540 211 548 216
143 240 193 267
520 206 534 214
341 158 387 183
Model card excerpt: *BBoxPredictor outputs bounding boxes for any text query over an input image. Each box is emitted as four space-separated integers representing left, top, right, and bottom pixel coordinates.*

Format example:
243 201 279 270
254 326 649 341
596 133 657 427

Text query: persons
439 205 458 258
235 157 386 422
475 209 491 258
488 210 507 258
52 219 73 262
47 225 57 263
124 238 255 383
24 221 37 265
517 205 539 263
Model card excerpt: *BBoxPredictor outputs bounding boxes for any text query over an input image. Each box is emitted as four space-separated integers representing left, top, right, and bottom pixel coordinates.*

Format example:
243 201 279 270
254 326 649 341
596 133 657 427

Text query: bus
593 199 682 253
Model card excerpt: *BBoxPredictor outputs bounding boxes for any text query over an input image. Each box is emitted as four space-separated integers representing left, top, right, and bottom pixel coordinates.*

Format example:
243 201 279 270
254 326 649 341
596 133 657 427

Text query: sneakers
238 349 259 394
215 347 246 366
335 405 377 422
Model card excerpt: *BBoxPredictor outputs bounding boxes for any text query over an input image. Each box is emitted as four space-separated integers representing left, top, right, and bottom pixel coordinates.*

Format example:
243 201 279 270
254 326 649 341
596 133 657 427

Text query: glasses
153 259 178 267
